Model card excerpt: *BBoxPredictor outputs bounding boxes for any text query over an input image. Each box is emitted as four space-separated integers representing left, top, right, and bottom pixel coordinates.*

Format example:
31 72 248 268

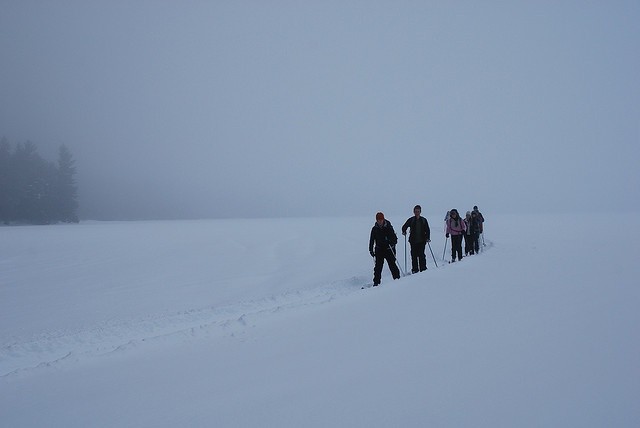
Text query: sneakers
421 268 426 271
412 269 416 273
373 283 377 286
458 258 461 260
452 258 455 261
394 276 398 279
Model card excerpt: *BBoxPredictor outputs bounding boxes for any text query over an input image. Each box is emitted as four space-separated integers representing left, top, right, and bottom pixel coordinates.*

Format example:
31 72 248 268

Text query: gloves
392 245 396 255
370 252 376 257
463 231 466 235
446 234 449 238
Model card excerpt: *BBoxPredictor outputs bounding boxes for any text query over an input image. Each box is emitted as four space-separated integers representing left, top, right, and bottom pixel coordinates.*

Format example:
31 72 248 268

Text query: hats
414 205 421 212
449 209 458 215
466 211 471 216
376 213 385 220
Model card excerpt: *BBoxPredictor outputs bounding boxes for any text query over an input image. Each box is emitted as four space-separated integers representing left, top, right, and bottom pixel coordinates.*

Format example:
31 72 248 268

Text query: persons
464 210 480 256
368 211 400 286
473 206 484 234
446 208 468 262
401 204 430 274
444 211 449 231
